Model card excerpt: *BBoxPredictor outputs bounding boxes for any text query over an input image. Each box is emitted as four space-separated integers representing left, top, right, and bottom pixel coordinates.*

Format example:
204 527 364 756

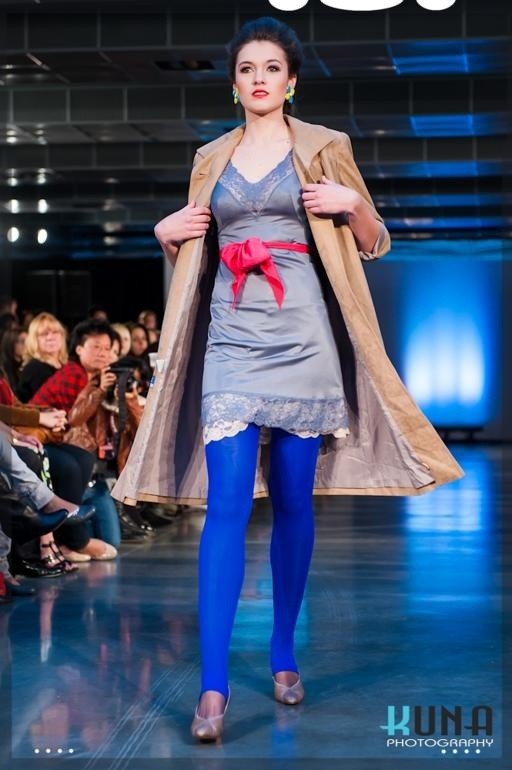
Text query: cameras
134 359 154 382
107 369 134 392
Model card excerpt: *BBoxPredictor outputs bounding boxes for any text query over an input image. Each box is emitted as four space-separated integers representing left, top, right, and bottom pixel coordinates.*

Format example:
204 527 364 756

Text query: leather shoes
3 500 117 595
190 683 231 740
271 672 305 706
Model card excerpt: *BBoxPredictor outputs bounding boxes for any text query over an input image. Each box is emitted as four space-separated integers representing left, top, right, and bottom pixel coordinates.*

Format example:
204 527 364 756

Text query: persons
112 16 466 740
0 300 189 605
